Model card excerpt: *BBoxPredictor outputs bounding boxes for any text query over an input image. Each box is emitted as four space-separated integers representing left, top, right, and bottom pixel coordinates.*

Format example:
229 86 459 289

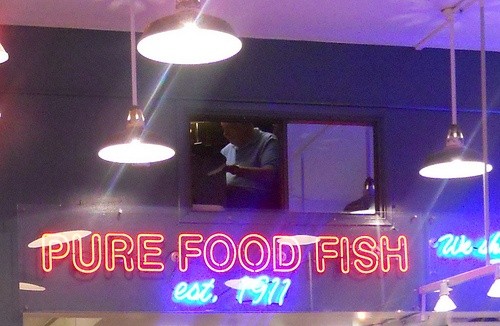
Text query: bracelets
233 165 239 174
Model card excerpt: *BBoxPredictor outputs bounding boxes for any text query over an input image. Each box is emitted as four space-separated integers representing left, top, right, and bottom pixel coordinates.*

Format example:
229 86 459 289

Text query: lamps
419 8 494 179
136 0 242 65
97 0 175 164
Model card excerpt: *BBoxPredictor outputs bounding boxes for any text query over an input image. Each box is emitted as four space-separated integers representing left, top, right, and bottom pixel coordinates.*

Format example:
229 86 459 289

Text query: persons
219 120 284 211
